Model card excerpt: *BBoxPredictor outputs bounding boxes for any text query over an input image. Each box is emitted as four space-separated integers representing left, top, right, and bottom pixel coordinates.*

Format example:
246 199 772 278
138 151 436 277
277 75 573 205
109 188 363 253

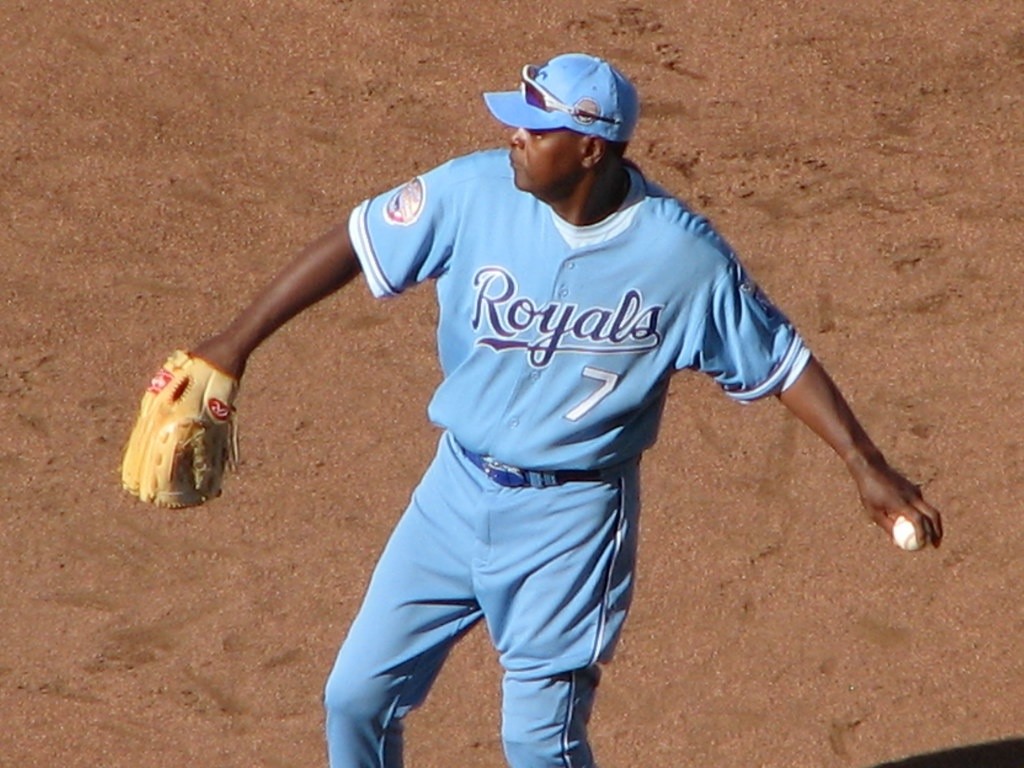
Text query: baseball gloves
117 344 242 514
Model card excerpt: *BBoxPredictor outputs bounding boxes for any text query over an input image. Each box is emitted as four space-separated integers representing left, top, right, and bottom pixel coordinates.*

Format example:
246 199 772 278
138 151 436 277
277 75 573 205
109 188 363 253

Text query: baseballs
891 514 932 552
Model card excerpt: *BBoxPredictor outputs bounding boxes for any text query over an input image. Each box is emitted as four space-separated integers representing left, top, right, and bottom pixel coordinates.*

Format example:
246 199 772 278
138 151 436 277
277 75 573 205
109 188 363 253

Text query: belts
459 447 642 489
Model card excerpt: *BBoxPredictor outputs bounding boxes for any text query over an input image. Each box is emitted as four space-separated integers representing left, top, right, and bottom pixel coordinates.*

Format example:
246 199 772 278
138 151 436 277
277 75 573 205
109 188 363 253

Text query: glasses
521 65 620 126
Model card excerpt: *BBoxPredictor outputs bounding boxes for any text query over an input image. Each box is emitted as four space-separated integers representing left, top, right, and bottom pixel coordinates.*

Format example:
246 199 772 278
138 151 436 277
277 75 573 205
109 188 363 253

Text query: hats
483 54 638 141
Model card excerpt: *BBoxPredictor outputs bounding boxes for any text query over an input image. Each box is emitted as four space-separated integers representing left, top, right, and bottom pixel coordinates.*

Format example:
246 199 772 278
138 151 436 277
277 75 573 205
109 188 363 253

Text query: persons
122 54 944 768
